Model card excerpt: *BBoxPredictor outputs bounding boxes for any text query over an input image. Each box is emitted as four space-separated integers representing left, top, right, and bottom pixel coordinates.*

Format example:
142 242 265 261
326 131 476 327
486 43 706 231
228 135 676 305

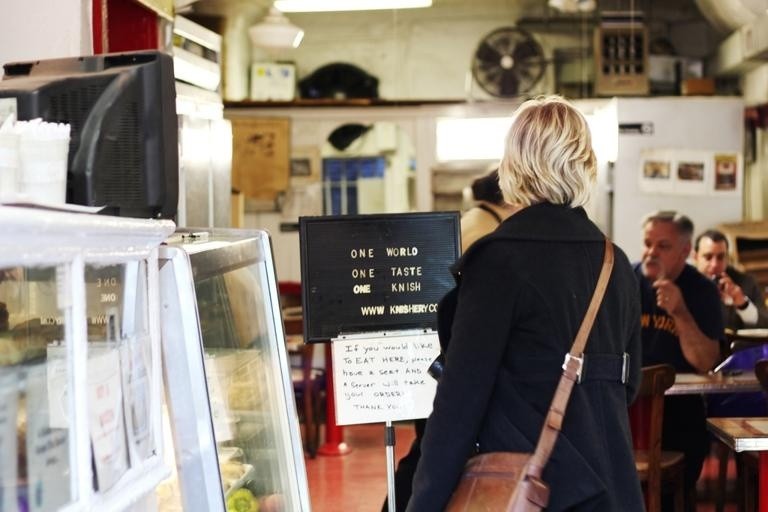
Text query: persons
624 209 731 505
401 92 652 512
377 166 531 511
688 229 768 329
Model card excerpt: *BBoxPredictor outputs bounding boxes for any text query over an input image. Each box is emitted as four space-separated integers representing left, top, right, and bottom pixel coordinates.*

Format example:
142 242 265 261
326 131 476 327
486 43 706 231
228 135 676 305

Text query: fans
467 22 557 101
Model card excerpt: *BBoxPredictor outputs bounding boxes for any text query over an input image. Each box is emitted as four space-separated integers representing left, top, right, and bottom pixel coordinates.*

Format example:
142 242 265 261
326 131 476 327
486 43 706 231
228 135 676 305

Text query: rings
663 295 671 305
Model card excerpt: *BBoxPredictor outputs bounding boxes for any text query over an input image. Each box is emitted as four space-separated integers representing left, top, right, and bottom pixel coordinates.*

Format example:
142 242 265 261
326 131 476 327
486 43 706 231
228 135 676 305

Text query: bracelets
736 295 749 310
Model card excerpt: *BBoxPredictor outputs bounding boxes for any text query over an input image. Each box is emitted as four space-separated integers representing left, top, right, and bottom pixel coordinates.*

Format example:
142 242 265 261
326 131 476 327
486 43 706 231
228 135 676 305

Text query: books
702 413 768 454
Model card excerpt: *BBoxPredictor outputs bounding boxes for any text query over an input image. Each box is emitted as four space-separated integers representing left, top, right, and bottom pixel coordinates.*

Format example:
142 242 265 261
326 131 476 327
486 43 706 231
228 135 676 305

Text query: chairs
754 357 768 395
628 363 686 512
281 293 326 459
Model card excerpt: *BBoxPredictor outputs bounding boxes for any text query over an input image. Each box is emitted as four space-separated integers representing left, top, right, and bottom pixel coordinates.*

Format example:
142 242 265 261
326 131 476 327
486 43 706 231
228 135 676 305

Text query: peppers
227 487 259 512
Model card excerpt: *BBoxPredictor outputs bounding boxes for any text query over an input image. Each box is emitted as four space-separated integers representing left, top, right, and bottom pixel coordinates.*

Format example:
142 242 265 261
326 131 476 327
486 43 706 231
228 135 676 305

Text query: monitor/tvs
0 48 179 226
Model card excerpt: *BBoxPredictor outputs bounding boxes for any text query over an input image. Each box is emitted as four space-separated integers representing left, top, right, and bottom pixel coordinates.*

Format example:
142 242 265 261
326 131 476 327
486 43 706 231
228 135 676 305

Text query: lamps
248 5 305 49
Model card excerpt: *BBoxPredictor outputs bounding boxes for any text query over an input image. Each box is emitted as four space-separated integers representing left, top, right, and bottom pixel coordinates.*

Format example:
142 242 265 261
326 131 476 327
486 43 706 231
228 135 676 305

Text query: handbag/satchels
444 451 551 512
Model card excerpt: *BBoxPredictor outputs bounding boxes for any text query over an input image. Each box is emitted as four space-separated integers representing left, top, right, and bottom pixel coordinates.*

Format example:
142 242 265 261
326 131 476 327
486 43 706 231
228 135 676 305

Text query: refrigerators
159 226 317 512
